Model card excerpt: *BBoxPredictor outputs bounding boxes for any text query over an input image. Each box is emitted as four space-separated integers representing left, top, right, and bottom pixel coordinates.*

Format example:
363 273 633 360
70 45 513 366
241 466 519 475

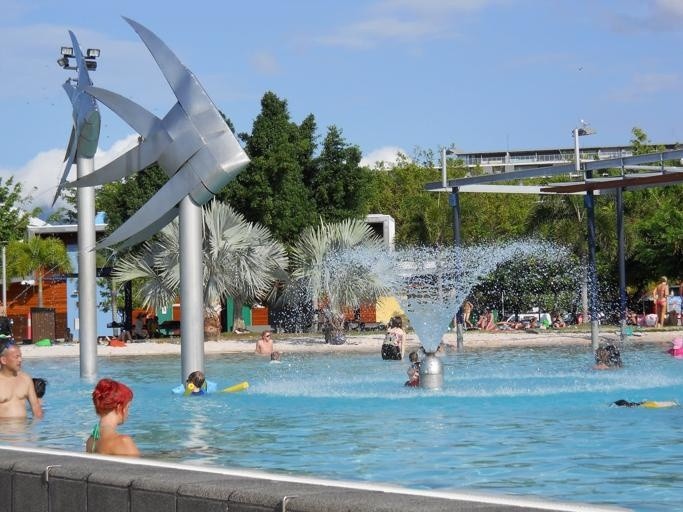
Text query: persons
0 337 43 421
62 327 73 343
268 350 281 364
254 330 274 353
408 352 419 366
185 371 206 396
96 311 167 346
379 315 406 360
402 362 421 388
86 377 140 455
31 377 48 404
437 276 683 370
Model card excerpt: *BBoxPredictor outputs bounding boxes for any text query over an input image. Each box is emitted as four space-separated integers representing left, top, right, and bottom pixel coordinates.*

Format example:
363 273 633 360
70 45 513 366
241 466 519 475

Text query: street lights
58 47 100 379
1 240 10 318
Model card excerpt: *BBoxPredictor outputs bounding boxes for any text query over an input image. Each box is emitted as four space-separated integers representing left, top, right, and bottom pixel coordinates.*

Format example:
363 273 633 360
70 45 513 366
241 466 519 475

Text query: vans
507 313 552 325
